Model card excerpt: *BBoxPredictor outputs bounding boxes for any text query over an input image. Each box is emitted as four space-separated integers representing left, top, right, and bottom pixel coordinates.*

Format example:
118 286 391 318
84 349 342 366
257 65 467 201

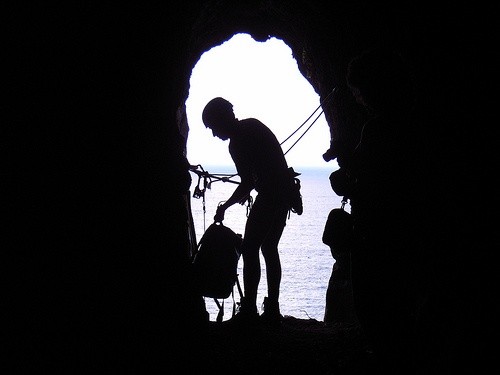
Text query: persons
203 97 295 325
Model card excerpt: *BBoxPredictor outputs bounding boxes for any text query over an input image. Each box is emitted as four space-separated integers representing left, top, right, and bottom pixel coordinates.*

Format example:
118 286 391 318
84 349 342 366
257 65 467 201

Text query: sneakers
225 297 281 322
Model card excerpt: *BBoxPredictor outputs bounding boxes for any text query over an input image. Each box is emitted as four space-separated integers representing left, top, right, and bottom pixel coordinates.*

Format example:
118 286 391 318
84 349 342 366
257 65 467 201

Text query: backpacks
188 220 243 300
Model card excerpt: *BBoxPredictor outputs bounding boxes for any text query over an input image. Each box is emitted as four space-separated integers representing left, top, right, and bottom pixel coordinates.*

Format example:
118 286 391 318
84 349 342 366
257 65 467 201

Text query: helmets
202 97 233 128
